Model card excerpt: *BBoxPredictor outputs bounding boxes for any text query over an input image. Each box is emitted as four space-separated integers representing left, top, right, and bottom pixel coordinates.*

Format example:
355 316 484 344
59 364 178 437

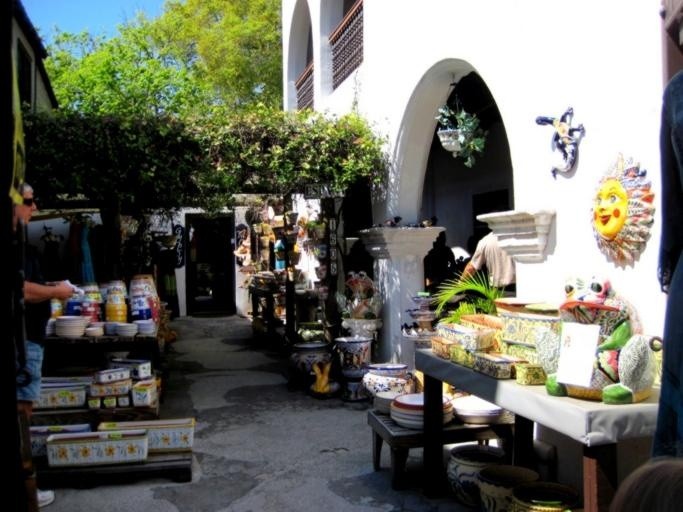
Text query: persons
460 232 517 292
10 183 77 509
609 459 683 511
274 228 287 318
424 231 455 329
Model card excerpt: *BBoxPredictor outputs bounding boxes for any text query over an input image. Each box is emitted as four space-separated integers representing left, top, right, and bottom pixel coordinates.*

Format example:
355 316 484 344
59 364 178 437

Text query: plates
390 392 456 430
86 321 138 337
374 391 404 412
55 316 90 339
133 320 156 335
45 319 56 336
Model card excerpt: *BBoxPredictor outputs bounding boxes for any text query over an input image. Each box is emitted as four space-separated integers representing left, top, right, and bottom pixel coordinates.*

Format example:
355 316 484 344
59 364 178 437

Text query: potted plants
435 105 489 170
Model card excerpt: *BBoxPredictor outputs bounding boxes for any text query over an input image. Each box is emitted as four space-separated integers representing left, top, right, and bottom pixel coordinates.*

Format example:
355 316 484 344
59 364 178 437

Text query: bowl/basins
105 287 128 323
100 279 129 300
129 274 162 323
451 396 502 423
50 283 103 320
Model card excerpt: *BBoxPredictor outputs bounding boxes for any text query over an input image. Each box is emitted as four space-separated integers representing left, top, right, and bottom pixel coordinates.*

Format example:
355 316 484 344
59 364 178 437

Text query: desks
249 283 305 329
367 409 515 491
415 347 660 512
36 328 166 374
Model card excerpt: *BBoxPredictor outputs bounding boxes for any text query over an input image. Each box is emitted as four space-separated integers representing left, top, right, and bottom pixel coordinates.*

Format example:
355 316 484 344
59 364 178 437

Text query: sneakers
37 489 55 508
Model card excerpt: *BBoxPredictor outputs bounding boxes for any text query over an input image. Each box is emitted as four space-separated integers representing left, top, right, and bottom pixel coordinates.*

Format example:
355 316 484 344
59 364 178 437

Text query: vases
342 367 370 403
361 362 414 407
291 342 332 390
446 444 506 501
507 480 576 511
474 463 539 511
297 320 324 343
431 294 560 385
333 336 374 369
342 317 383 340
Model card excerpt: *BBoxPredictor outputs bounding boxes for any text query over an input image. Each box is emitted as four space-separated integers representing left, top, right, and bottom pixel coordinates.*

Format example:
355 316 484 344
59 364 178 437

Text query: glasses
23 197 33 206
